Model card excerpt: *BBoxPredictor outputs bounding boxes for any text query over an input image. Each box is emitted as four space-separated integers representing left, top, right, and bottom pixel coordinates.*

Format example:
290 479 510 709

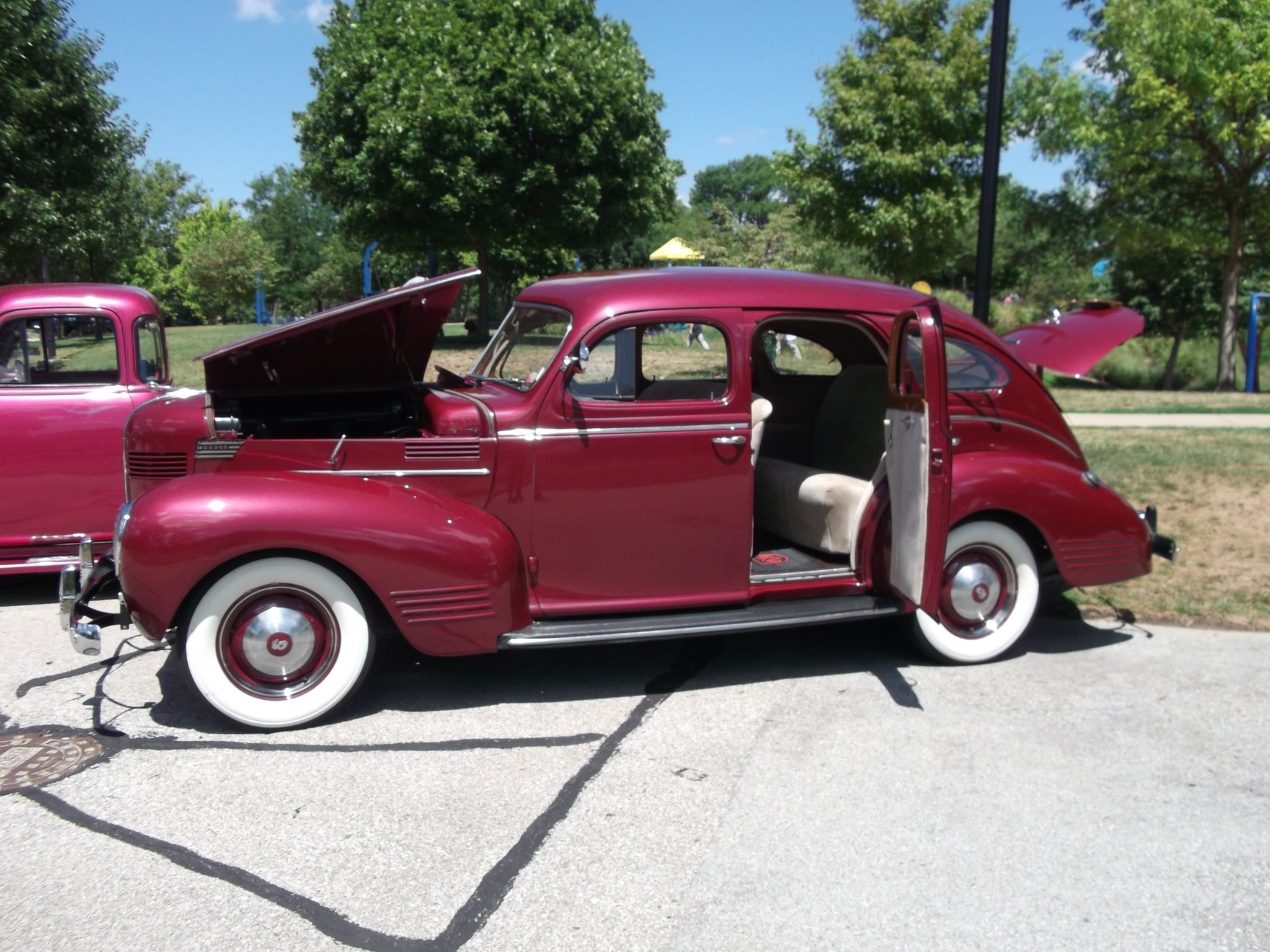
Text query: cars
59 261 1179 736
0 281 232 580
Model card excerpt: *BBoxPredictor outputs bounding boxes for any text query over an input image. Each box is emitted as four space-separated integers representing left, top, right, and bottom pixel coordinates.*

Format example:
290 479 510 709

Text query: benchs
755 360 877 553
646 382 773 470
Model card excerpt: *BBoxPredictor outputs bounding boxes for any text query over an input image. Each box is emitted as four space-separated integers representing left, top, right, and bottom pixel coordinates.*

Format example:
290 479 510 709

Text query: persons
646 323 684 340
1004 293 1013 305
1093 259 1113 278
775 334 802 360
687 323 710 350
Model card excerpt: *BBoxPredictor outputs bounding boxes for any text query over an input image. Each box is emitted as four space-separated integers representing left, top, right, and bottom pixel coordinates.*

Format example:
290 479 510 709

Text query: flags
363 241 378 297
